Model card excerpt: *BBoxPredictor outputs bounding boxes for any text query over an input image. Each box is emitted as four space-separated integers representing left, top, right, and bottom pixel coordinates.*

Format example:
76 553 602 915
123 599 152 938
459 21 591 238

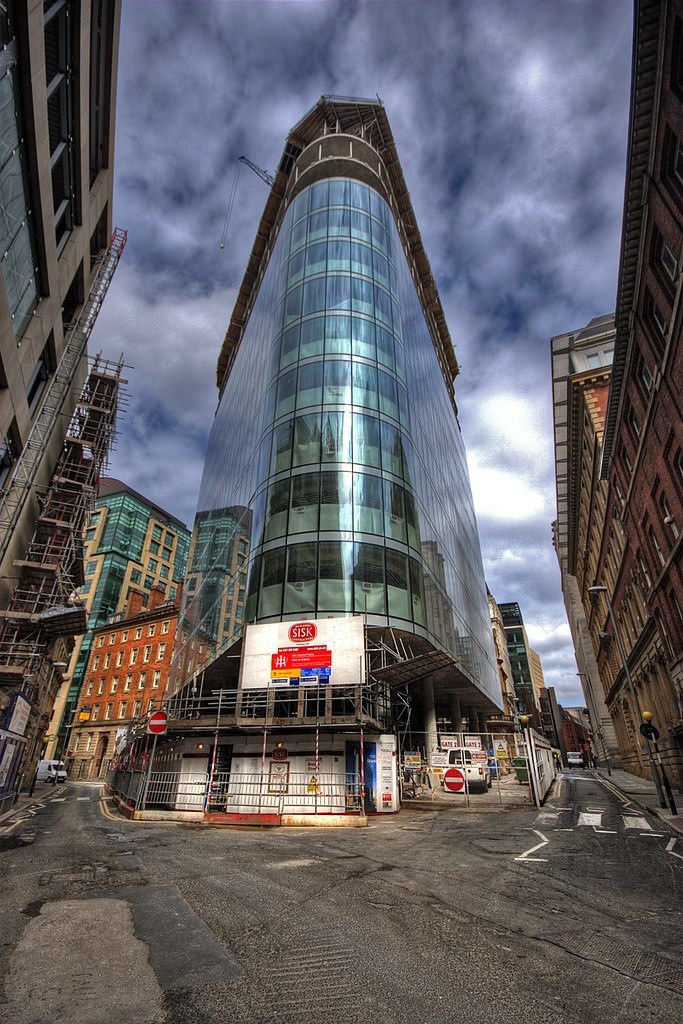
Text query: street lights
521 714 529 727
576 671 611 776
28 736 49 797
643 710 678 817
574 707 593 769
586 584 668 809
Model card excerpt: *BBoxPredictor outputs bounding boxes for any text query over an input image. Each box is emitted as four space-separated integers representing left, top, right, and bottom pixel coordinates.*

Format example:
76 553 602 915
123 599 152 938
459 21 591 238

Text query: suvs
567 751 585 770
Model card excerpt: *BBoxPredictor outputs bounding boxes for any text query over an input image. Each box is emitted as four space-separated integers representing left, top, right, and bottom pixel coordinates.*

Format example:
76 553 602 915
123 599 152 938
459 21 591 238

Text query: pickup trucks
443 747 493 794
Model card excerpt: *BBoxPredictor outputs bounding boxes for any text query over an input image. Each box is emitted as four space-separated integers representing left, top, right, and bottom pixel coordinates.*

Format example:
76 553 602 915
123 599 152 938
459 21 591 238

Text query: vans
35 760 68 784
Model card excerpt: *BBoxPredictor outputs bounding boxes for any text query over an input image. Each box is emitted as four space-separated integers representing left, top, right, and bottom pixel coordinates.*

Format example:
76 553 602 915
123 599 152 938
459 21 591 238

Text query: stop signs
148 711 168 735
444 768 465 793
